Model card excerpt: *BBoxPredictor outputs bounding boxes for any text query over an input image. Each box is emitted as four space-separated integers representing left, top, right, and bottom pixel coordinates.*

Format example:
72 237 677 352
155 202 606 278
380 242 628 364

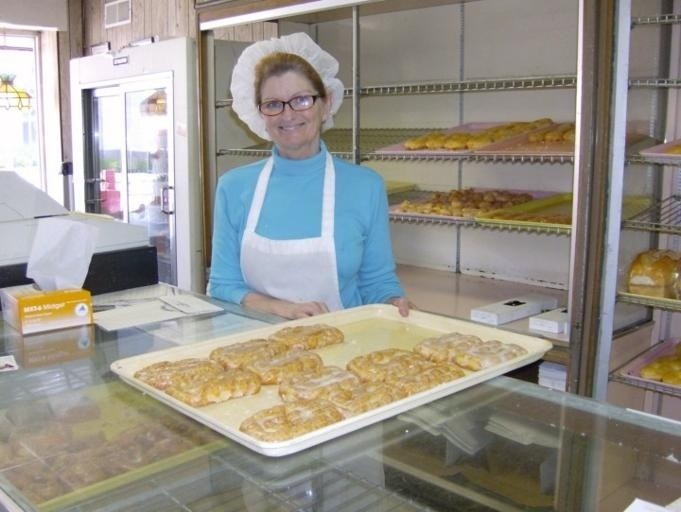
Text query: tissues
1 216 99 336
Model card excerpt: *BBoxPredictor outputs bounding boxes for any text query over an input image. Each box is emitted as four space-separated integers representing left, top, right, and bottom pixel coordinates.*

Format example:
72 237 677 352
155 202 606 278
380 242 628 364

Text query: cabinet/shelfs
0 284 681 512
579 1 681 512
70 39 203 294
194 1 608 512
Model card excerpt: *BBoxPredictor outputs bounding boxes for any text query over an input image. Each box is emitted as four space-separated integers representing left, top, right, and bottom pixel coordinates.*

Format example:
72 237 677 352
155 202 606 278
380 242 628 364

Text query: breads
404 114 552 151
135 318 527 447
643 355 681 386
401 185 532 215
674 341 681 355
626 249 681 304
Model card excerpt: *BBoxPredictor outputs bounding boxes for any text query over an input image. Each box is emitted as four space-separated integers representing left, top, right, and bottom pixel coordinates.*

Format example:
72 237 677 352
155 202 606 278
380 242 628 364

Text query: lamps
0 74 33 108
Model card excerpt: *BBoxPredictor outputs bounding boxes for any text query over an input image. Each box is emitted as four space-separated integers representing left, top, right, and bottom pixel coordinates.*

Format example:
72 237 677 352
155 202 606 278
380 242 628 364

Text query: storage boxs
0 281 93 335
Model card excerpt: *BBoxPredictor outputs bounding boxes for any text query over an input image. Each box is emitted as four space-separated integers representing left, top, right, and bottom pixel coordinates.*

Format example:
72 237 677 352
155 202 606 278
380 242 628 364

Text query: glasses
258 94 321 116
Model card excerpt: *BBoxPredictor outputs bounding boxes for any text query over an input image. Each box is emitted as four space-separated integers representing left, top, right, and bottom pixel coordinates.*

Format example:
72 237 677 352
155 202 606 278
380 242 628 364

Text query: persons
207 32 409 319
